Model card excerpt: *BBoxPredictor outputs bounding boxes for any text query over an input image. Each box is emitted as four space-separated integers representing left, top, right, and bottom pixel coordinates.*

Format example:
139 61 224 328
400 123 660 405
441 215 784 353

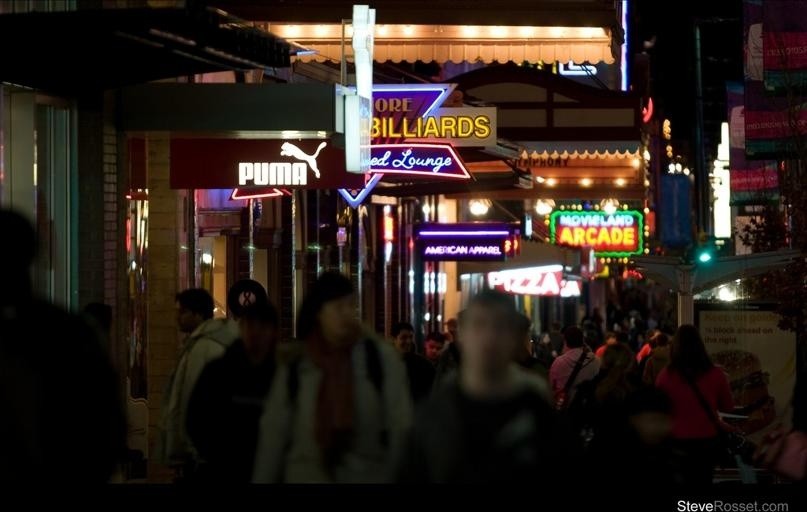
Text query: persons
156 271 807 495
1 204 129 490
